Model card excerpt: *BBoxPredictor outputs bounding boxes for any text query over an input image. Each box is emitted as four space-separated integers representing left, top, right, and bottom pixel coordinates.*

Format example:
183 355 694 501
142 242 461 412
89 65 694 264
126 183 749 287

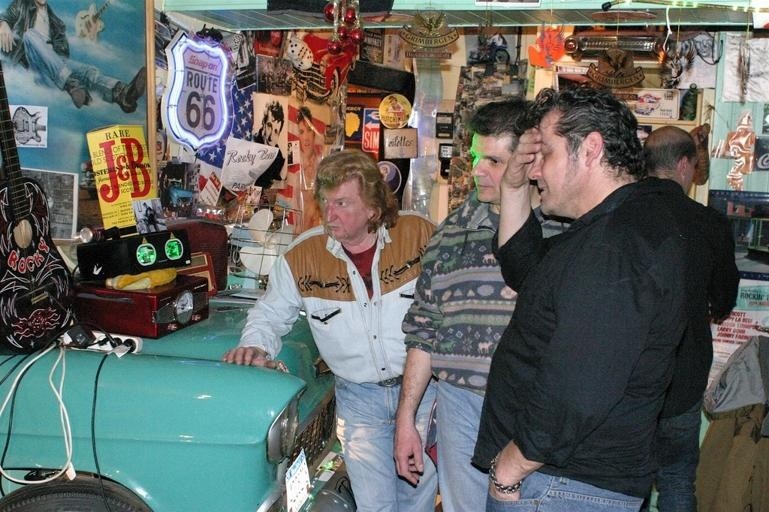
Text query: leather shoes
69 84 93 109
120 67 146 113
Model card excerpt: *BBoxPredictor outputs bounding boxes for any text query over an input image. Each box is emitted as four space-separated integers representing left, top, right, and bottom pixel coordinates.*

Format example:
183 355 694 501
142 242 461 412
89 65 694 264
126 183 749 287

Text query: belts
378 375 403 388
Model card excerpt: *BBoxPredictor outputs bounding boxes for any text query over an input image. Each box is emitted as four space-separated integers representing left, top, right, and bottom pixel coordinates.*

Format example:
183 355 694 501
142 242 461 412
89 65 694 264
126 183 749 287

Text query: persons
294 108 326 183
225 148 445 511
0 0 146 113
470 85 711 511
392 101 577 512
644 126 699 202
252 102 287 193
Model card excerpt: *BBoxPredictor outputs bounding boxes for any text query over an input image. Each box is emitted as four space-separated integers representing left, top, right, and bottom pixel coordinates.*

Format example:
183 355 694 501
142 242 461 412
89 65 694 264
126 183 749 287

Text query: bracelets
486 459 528 495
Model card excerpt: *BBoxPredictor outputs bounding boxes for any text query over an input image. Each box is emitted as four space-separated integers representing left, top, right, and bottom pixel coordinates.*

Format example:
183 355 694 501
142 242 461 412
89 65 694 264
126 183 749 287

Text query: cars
1 309 337 510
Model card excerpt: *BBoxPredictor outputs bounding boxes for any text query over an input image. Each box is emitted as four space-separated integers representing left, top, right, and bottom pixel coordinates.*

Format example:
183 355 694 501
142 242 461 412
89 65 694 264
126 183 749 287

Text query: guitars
0 60 75 355
